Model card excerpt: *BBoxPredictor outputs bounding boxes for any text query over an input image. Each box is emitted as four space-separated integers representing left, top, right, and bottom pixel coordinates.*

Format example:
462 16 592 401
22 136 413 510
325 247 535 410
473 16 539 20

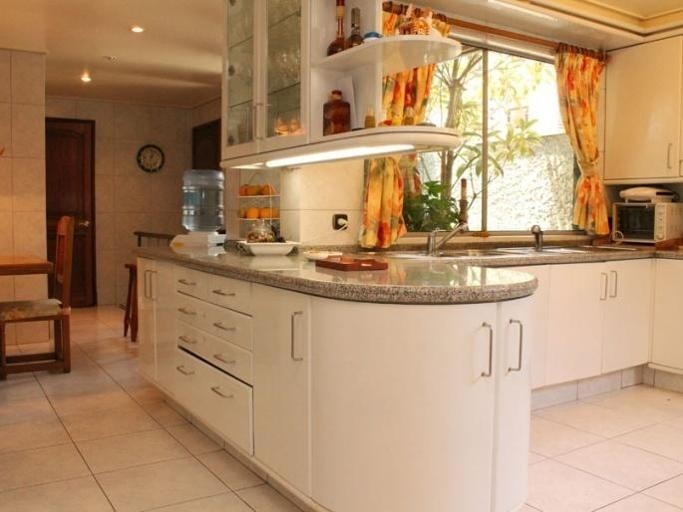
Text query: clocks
137 144 165 173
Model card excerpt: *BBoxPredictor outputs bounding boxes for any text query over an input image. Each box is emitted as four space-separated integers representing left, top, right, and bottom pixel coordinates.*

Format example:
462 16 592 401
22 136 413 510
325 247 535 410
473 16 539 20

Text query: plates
303 250 344 261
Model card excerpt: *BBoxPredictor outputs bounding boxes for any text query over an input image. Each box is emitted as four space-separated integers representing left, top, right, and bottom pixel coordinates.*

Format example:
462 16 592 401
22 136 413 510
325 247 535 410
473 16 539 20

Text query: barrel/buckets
181 170 225 233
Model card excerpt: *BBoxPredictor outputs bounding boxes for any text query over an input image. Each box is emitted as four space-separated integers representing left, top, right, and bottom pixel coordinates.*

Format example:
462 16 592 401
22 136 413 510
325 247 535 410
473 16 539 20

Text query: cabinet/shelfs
176 265 254 456
221 0 312 161
310 0 462 144
313 294 536 512
252 282 312 498
137 256 177 396
604 35 683 179
546 259 651 386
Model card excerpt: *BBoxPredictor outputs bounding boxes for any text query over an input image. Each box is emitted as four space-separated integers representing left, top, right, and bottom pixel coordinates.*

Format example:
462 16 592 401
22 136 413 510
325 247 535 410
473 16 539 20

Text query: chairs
0 216 75 380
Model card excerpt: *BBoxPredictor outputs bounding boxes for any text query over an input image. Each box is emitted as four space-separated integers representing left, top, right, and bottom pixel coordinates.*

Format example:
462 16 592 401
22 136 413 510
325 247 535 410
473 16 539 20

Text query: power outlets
333 214 348 230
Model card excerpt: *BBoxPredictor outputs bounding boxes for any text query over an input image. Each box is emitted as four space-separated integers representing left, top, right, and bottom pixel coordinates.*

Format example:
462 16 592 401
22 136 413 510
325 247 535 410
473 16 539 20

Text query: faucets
426 224 469 253
531 225 543 253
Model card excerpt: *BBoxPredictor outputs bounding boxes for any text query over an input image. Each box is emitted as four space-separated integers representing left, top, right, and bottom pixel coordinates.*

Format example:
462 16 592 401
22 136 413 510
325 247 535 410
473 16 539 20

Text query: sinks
428 246 525 259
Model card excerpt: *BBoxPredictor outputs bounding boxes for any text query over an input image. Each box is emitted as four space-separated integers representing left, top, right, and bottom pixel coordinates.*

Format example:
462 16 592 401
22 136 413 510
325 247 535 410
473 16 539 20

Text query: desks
0 255 54 275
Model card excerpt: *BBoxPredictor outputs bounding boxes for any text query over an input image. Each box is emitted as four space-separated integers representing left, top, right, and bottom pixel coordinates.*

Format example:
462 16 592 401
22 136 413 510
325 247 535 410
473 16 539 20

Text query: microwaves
610 201 683 244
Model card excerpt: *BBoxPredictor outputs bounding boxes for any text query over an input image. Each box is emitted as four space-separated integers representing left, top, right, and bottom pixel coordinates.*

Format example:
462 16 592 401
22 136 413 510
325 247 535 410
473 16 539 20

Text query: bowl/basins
249 256 302 274
238 240 300 256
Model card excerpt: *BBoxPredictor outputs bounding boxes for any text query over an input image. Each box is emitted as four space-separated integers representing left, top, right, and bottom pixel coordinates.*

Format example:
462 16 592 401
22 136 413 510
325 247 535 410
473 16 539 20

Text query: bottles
402 106 415 126
326 0 365 55
322 88 351 136
364 107 375 129
246 217 278 243
